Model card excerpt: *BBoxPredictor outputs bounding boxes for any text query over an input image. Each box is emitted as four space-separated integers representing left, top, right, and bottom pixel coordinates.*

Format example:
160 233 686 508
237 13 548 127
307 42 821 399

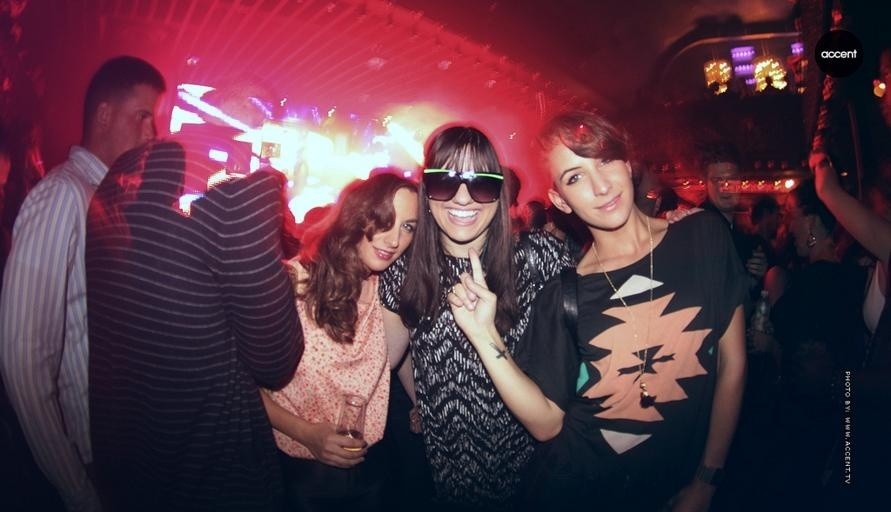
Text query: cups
334 393 369 453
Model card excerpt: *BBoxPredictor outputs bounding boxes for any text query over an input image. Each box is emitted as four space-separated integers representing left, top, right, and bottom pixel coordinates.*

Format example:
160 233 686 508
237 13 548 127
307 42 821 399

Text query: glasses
422 169 503 206
